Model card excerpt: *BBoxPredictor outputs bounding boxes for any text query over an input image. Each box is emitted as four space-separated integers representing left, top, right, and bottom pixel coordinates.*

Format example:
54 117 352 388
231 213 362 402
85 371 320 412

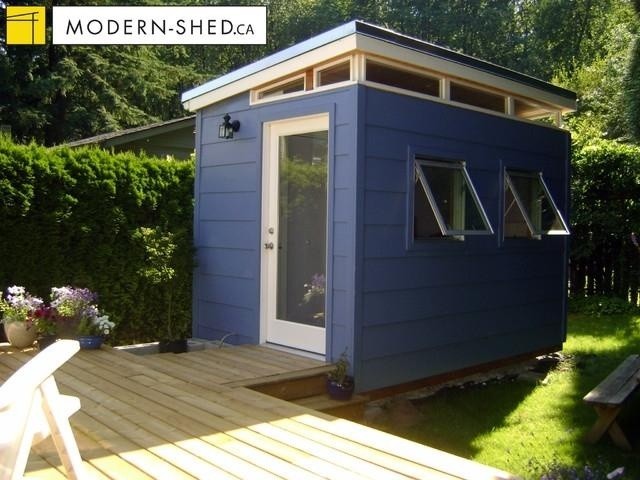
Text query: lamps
219 114 241 138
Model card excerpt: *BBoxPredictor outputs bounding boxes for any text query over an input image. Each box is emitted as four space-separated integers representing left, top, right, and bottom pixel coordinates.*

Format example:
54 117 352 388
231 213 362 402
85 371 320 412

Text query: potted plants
328 346 354 400
135 227 200 354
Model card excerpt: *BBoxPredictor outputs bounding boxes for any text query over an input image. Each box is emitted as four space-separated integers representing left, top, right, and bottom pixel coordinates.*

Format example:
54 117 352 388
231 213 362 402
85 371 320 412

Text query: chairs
0 339 87 480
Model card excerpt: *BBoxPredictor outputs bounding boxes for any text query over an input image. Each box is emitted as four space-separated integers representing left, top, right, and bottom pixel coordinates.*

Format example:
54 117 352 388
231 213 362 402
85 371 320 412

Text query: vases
4 320 102 349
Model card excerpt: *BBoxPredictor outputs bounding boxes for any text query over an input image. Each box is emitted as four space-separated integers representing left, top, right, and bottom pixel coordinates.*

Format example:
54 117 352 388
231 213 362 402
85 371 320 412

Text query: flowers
0 285 117 334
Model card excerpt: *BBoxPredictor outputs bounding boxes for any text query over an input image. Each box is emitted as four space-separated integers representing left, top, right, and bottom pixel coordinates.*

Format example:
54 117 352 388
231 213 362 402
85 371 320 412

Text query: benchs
582 353 638 454
